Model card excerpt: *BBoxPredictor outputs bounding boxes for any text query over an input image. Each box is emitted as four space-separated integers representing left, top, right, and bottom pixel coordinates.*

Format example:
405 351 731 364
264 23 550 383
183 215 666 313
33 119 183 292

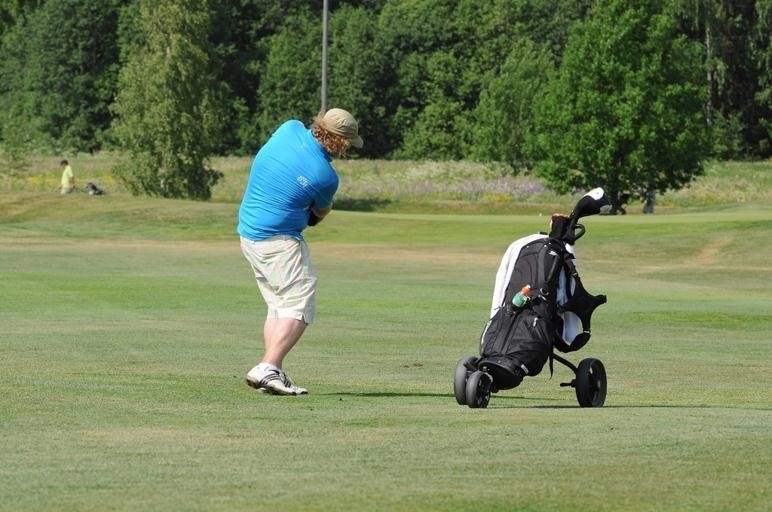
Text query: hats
322 107 364 148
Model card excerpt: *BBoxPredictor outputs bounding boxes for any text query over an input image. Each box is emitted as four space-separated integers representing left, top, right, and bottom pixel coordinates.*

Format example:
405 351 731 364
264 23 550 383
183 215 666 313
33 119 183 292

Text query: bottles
512 284 530 308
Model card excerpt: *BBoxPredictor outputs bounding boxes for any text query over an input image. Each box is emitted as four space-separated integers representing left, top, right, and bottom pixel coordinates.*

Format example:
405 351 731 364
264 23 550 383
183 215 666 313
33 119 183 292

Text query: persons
56 159 76 195
638 175 657 214
235 104 367 396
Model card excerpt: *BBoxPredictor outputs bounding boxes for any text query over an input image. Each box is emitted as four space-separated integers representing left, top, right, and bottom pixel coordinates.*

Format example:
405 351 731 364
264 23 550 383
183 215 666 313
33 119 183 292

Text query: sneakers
246 364 308 394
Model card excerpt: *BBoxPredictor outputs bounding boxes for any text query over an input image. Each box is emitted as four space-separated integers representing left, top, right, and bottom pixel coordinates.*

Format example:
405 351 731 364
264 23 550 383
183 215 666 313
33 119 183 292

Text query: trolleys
453 181 615 411
